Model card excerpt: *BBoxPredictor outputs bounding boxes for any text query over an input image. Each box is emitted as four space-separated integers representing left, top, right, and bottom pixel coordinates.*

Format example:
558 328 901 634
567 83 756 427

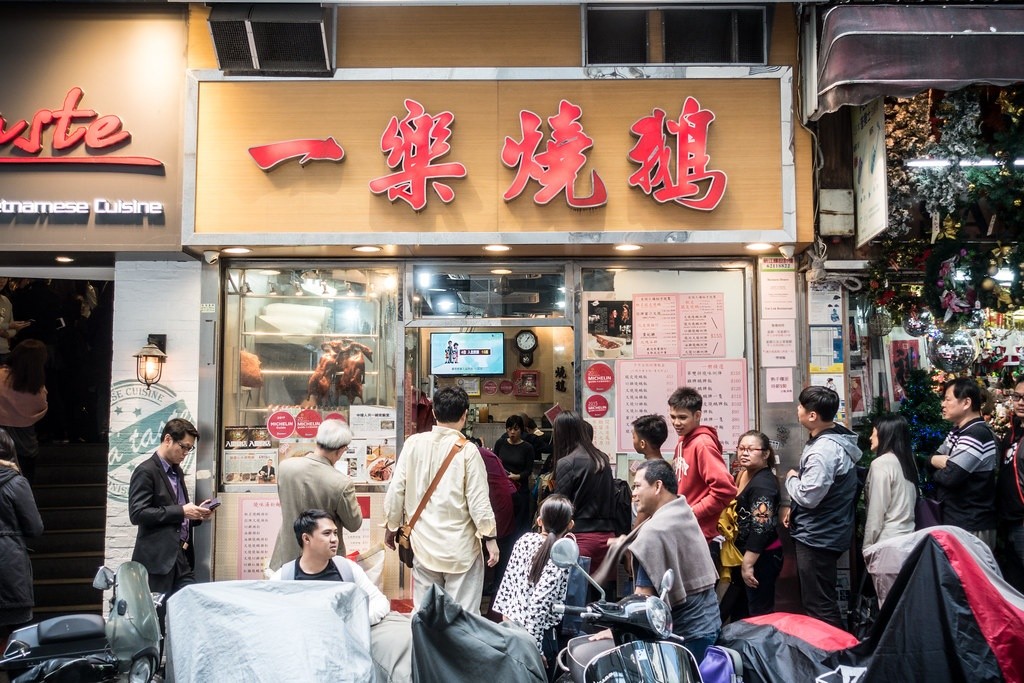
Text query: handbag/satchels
562 532 592 635
912 451 939 531
395 525 414 568
612 479 635 536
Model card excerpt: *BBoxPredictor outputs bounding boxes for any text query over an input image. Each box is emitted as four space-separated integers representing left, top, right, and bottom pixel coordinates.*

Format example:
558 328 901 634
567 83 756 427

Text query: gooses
336 343 374 404
306 339 342 405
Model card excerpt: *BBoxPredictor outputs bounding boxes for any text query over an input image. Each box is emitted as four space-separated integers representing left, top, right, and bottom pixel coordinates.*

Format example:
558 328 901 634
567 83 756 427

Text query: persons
267 419 364 577
259 459 275 478
0 275 114 553
270 508 390 626
0 428 43 683
463 375 1024 683
383 386 500 618
128 418 216 670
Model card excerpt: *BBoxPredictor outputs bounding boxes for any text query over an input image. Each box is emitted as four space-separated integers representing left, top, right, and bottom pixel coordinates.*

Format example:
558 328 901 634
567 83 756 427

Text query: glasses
735 444 765 454
176 441 195 452
1011 392 1024 401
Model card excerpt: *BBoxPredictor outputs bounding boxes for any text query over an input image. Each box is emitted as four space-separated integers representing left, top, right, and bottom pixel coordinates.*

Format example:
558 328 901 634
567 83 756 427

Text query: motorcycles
0 560 163 683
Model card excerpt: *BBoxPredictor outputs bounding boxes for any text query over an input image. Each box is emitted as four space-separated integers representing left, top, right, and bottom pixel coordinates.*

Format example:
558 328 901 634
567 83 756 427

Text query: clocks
515 329 538 367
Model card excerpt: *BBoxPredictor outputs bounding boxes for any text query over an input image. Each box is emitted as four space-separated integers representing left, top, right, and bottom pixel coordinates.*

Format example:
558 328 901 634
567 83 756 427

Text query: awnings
810 4 1024 122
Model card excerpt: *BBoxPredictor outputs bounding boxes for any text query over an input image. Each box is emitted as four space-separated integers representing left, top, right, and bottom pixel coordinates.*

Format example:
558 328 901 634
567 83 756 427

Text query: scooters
548 537 706 683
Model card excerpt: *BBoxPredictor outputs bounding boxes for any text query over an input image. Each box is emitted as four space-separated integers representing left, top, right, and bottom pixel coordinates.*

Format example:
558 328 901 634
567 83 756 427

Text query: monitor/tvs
430 332 505 377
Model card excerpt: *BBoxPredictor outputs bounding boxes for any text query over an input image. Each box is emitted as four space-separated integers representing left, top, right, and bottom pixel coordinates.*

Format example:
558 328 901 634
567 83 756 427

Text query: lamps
132 334 167 389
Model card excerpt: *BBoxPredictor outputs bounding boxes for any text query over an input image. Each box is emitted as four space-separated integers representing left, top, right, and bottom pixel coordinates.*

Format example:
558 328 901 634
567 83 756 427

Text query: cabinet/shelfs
237 286 381 426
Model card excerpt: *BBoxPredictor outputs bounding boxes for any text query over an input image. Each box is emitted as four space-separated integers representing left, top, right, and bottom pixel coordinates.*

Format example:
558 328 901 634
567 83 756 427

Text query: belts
180 542 188 550
